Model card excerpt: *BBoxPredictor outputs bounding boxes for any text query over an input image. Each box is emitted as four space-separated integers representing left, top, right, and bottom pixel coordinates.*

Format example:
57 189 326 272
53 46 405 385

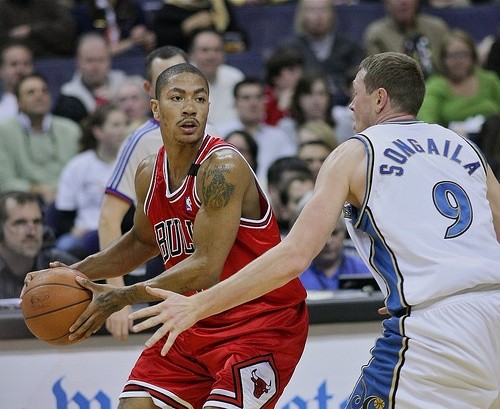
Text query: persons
360 0 453 76
215 76 297 198
294 120 338 184
279 175 315 240
96 45 191 341
129 52 500 409
263 43 305 126
20 64 309 409
475 33 500 73
297 212 372 291
266 157 309 238
225 130 258 174
416 29 500 137
279 0 365 105
275 71 338 137
0 0 247 298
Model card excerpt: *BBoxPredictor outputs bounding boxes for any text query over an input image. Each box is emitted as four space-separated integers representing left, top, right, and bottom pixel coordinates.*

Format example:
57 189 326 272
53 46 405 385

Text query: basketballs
18 265 95 347
